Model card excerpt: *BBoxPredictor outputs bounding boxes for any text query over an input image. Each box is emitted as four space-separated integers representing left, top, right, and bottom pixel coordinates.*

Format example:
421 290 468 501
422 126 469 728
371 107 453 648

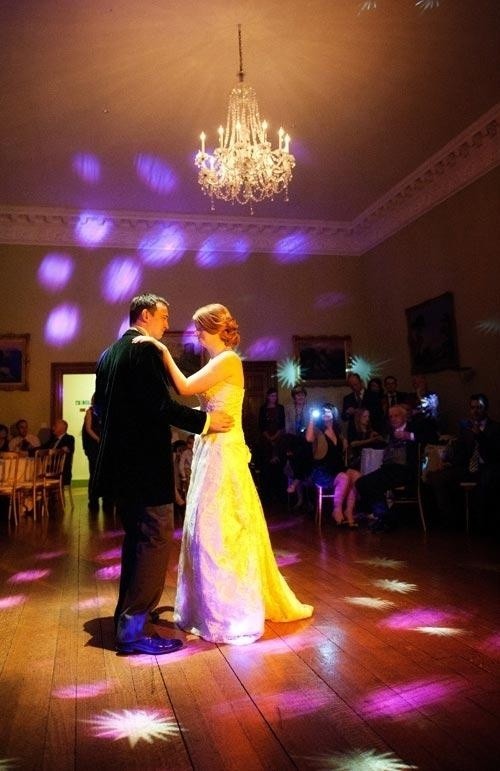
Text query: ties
356 391 362 401
390 394 396 407
50 438 58 448
467 439 480 472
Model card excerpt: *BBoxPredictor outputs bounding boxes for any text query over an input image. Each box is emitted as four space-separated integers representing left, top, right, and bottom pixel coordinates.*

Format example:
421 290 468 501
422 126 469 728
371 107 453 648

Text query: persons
91 292 235 650
132 301 271 648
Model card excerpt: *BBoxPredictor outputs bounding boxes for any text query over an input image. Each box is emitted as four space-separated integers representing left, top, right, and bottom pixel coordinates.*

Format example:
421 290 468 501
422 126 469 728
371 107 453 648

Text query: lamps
193 19 298 215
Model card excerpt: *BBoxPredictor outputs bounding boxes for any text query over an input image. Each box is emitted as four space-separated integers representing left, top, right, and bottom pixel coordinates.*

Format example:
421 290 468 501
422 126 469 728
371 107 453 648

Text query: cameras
374 432 385 437
311 409 325 417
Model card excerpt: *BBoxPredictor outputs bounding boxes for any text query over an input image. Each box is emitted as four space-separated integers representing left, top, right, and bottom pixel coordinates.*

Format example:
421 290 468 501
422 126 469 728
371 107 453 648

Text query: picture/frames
0 329 33 395
405 290 463 378
159 329 208 389
289 332 358 391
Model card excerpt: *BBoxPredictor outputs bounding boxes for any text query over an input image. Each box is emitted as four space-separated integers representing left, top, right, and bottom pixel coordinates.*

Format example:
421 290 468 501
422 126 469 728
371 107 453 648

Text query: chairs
170 438 500 533
0 425 77 525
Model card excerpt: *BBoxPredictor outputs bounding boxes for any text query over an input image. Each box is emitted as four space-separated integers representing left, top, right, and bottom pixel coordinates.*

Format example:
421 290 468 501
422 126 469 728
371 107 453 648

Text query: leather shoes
116 632 182 654
